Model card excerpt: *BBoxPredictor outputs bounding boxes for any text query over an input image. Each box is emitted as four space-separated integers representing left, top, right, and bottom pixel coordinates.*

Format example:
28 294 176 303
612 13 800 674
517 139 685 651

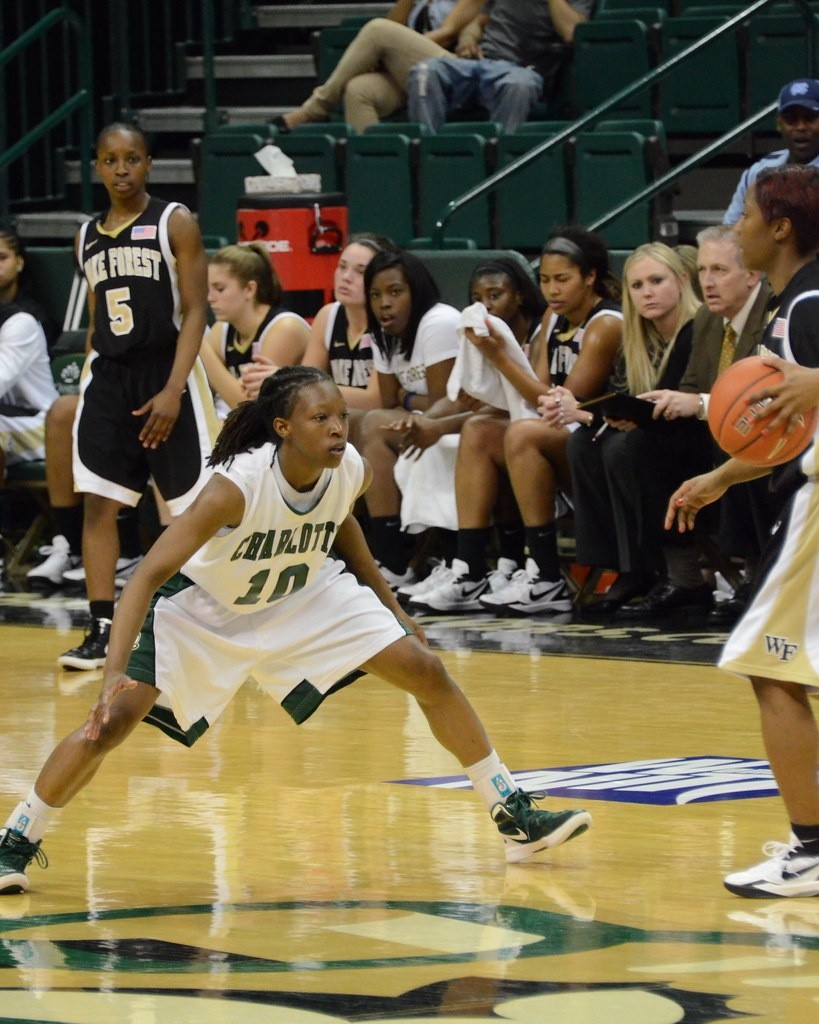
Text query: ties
717 324 737 377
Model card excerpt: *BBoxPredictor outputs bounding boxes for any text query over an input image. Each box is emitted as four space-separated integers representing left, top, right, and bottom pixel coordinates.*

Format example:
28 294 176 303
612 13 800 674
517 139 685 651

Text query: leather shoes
615 581 718 624
708 589 757 624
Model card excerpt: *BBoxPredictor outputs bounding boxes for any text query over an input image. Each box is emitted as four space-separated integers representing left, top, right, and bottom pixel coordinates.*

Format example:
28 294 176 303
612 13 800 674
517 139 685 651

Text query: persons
722 79 819 225
56 125 209 671
0 366 593 895
0 229 60 577
29 225 804 622
638 166 819 896
749 358 819 435
268 0 596 137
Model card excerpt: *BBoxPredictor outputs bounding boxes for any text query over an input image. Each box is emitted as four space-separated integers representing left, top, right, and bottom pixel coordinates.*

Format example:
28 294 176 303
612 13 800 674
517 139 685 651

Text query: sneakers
397 559 451 597
490 787 592 863
727 902 819 966
505 865 596 923
375 565 419 590
409 558 492 612
724 831 819 897
0 827 48 894
57 617 112 671
479 557 573 614
26 534 84 585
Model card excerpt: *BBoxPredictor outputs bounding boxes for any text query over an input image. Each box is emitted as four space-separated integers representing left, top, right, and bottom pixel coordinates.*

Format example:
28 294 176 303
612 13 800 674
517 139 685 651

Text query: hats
779 79 819 112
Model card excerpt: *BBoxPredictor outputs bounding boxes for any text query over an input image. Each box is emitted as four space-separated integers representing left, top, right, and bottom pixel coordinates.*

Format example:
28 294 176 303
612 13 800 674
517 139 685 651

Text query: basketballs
707 355 819 469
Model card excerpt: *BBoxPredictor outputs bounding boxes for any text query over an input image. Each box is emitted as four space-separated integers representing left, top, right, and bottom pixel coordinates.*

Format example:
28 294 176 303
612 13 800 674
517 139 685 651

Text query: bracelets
403 391 416 411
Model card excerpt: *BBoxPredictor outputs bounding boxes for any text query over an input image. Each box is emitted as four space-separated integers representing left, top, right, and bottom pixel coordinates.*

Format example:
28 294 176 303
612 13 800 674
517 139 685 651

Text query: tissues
244 145 322 195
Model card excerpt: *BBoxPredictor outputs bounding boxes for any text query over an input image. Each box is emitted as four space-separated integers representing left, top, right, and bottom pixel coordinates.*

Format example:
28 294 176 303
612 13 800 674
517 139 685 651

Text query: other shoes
579 571 651 621
268 114 293 134
63 555 145 587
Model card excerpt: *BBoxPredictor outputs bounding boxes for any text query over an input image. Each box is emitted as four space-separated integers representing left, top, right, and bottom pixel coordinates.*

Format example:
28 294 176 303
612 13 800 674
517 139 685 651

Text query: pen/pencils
592 422 609 441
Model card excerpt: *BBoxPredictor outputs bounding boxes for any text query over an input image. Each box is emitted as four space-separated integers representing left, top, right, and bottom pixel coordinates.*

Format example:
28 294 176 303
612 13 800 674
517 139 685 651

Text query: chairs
0 0 819 588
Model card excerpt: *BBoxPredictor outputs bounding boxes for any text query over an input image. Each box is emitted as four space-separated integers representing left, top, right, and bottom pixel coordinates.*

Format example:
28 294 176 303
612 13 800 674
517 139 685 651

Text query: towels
393 432 459 534
447 302 581 432
